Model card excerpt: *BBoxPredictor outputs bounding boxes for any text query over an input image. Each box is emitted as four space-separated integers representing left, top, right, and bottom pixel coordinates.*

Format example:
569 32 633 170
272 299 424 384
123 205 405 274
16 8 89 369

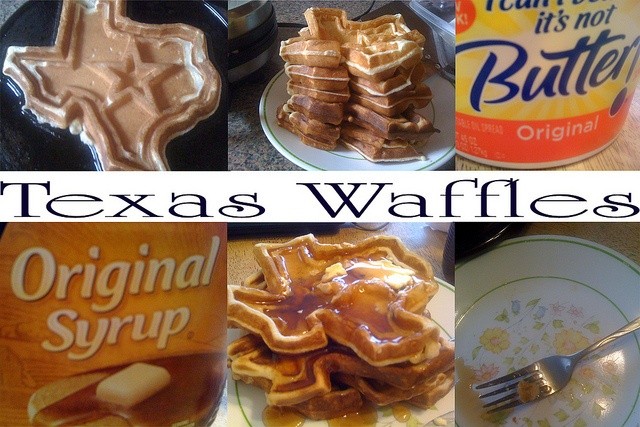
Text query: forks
475 318 640 414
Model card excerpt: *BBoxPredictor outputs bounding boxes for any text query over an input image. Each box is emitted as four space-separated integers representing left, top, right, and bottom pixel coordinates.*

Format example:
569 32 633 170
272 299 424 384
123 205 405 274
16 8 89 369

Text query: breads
226 233 455 422
276 5 441 165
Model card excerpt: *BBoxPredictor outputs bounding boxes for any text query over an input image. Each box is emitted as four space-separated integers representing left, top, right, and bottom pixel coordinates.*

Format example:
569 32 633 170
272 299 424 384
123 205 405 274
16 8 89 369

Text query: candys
96 361 173 410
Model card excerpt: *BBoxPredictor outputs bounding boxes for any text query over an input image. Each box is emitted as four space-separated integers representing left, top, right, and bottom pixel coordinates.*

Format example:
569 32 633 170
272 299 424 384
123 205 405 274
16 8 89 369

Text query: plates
260 62 455 170
455 233 640 426
227 277 455 426
